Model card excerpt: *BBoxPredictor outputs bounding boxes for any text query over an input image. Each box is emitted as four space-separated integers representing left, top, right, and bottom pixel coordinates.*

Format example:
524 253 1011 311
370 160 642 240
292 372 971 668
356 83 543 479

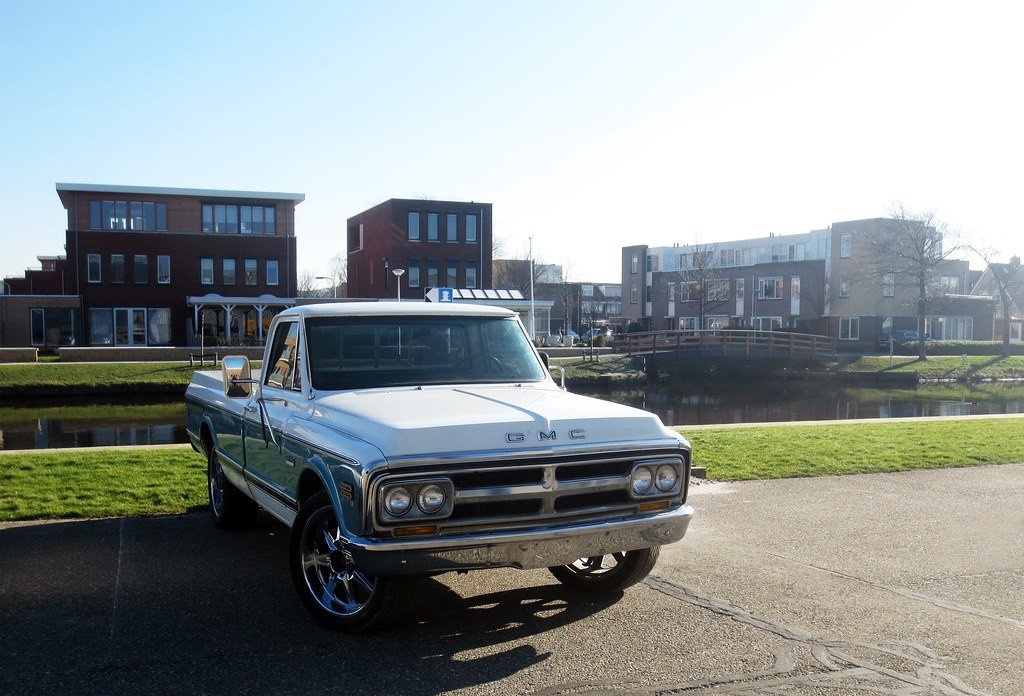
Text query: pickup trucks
184 301 695 636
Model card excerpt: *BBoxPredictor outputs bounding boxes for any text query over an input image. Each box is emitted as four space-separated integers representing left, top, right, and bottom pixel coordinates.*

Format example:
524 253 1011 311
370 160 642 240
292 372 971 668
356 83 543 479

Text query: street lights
392 268 405 356
316 276 337 300
752 289 760 343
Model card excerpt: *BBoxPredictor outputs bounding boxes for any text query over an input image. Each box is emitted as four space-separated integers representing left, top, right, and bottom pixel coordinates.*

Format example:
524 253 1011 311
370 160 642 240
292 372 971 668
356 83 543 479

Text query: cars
877 330 930 352
553 329 581 345
582 328 615 343
